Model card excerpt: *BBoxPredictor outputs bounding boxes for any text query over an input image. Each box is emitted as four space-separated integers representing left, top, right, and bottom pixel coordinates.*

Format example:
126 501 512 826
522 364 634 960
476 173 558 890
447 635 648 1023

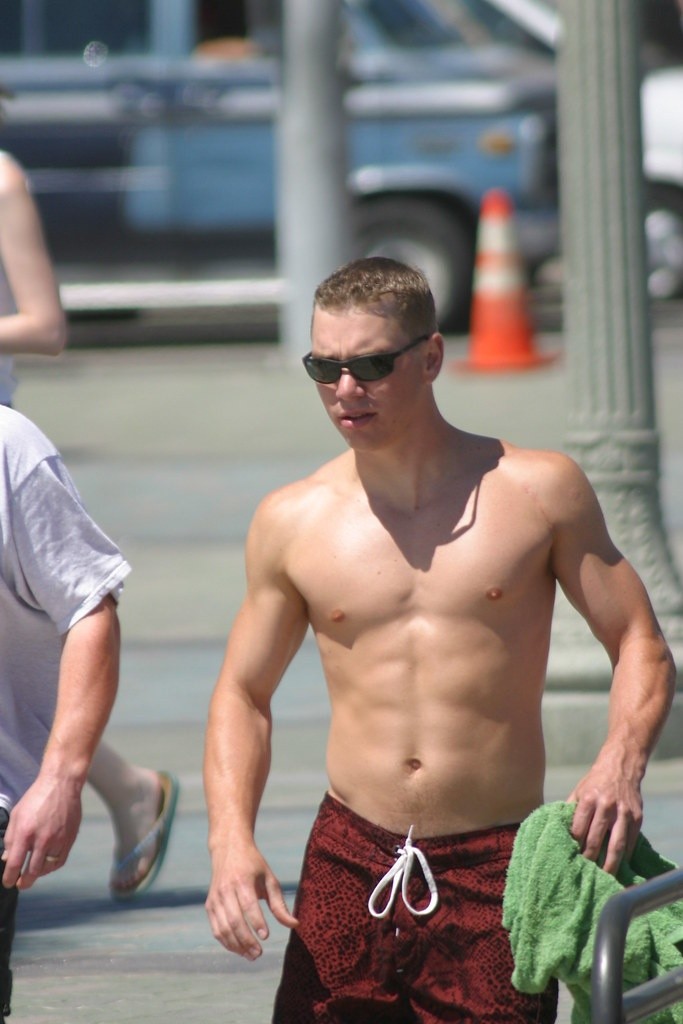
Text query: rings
46 856 58 862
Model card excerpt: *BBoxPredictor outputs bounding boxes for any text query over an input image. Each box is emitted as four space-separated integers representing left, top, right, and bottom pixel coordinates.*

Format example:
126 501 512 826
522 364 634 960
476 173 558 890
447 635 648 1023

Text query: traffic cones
451 187 557 374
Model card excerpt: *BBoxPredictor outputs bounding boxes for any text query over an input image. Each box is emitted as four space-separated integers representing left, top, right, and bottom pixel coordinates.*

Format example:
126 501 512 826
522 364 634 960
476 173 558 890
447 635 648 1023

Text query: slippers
110 771 180 900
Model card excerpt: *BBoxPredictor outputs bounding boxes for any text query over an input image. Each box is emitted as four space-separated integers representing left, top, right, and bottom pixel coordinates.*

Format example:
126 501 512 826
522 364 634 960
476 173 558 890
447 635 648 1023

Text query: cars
0 0 566 346
421 0 683 307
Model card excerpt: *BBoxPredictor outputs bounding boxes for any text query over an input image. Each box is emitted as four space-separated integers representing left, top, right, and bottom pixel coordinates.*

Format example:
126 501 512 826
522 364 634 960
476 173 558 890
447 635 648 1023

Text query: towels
500 800 682 1024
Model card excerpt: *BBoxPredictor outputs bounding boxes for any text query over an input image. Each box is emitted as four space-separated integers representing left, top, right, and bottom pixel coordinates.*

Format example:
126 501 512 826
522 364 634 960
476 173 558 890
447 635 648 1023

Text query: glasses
303 336 430 385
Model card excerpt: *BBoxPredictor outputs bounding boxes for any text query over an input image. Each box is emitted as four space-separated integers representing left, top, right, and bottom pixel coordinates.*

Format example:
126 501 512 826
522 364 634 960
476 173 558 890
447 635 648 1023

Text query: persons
0 151 181 906
204 256 675 1024
0 405 134 1024
